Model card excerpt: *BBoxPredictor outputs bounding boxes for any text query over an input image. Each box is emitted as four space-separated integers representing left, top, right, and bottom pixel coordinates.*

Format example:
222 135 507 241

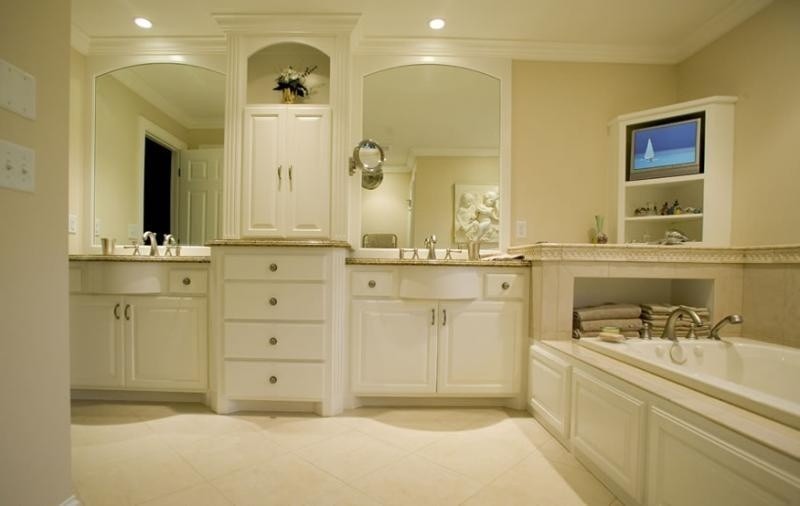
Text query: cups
102 236 116 255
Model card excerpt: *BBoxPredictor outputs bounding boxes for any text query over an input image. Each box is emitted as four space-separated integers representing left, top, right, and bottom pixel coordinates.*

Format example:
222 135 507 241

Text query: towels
571 302 712 341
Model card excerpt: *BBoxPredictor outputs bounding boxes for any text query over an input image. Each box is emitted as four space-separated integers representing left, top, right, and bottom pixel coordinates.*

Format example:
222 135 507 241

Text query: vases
282 88 295 104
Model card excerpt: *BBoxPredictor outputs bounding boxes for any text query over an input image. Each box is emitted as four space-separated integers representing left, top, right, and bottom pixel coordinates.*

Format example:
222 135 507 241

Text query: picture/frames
626 110 705 181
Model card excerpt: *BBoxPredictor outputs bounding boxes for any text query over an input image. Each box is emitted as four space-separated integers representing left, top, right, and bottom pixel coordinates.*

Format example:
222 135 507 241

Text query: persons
458 191 499 243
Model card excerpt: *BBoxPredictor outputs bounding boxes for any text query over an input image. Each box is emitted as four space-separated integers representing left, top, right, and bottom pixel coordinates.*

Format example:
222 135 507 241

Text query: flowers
273 61 318 98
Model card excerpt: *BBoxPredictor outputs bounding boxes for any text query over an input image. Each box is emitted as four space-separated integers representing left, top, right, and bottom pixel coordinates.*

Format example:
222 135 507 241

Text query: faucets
427 235 439 258
660 304 706 343
424 237 429 249
141 230 160 257
712 312 746 338
161 233 177 247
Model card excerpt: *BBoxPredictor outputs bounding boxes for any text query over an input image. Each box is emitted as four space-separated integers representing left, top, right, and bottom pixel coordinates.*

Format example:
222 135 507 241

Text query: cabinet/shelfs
346 263 532 411
605 94 738 247
240 106 331 241
209 245 349 418
69 259 213 406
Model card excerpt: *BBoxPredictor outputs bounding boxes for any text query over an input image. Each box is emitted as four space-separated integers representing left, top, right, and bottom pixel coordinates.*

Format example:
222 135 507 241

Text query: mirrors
350 138 385 174
359 61 502 252
88 60 226 248
360 169 383 190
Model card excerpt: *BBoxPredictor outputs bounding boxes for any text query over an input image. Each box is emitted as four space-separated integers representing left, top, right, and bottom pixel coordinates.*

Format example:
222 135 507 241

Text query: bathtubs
541 336 800 506
